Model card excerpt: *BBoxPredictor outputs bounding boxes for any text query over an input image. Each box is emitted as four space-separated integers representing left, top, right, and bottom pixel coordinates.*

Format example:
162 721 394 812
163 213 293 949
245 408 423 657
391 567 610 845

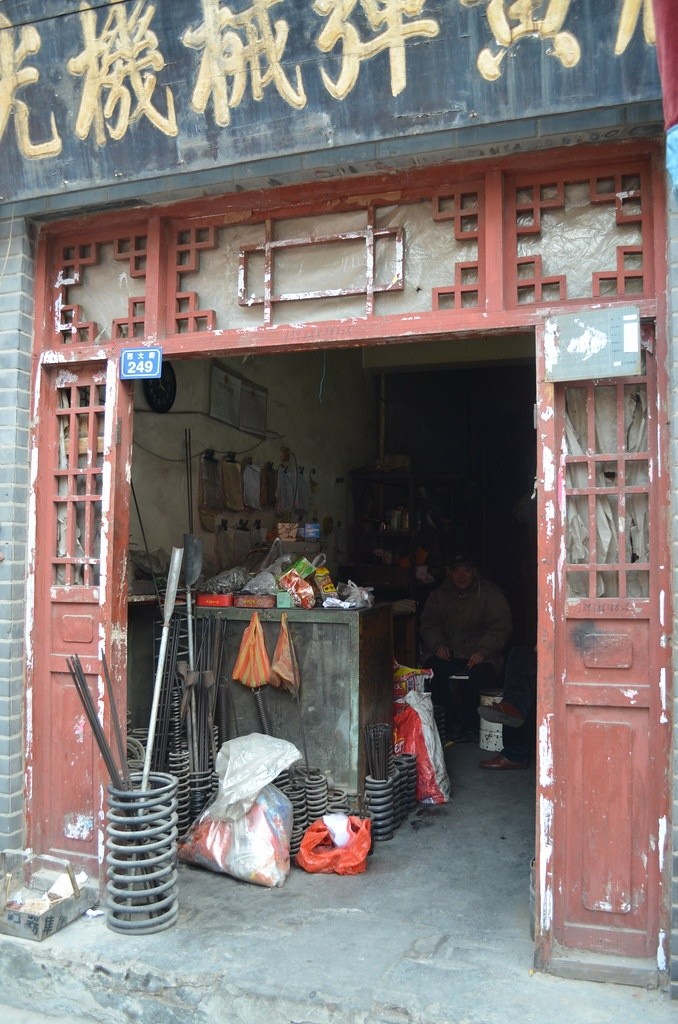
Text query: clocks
142 359 177 413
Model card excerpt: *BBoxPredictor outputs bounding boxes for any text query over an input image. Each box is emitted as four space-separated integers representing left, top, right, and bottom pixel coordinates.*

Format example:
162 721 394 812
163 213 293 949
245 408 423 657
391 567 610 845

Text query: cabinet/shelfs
349 470 459 669
175 601 395 804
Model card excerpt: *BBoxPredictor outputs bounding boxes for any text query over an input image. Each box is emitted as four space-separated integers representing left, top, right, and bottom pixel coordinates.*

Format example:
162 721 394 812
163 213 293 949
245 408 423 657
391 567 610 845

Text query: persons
476 640 536 770
419 553 514 747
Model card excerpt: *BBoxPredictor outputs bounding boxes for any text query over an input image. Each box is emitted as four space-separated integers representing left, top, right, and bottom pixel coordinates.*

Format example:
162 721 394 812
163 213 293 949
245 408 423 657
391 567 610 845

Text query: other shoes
477 701 525 728
478 752 529 770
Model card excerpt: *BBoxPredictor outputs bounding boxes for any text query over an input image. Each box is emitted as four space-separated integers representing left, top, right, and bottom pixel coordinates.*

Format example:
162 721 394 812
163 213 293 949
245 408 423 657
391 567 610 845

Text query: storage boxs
277 593 295 607
195 591 234 607
234 594 277 608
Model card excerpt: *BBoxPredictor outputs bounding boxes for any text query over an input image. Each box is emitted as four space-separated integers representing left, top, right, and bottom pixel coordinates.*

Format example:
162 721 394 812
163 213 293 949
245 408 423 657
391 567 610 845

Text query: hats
448 550 475 576
